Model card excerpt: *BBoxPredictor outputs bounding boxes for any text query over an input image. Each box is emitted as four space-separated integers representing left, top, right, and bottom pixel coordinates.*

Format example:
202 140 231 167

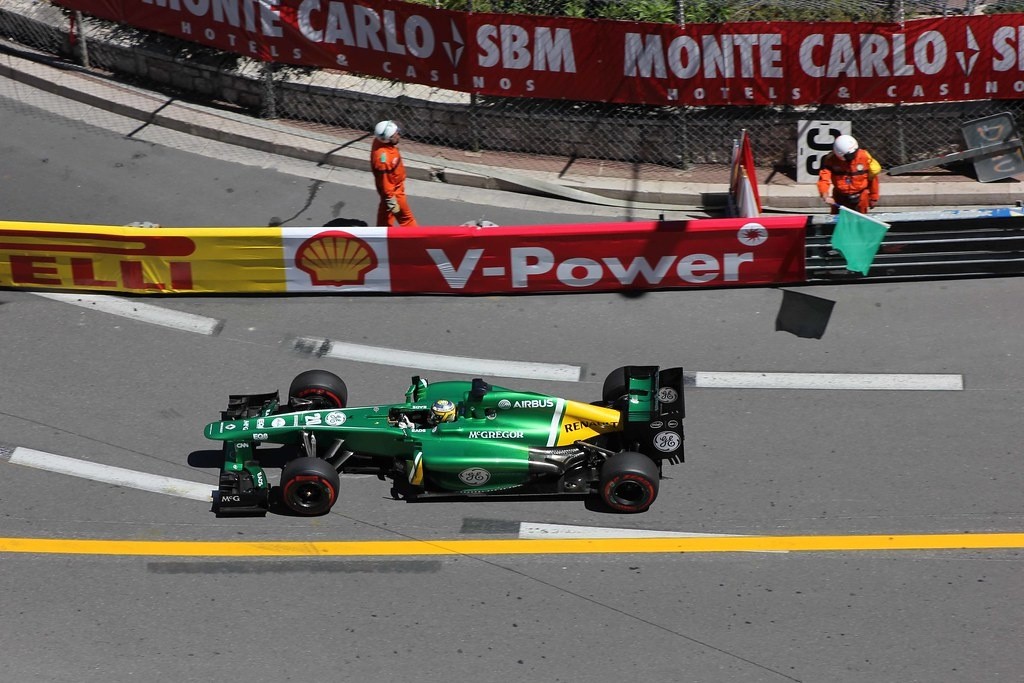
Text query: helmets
833 135 858 161
375 119 398 143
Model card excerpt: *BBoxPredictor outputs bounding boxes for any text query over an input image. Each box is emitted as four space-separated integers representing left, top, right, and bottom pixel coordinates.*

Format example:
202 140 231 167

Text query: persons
386 398 456 432
818 135 880 214
370 120 418 227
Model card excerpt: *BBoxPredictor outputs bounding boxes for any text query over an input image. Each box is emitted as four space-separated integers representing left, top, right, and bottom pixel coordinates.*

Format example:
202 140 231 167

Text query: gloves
385 197 400 213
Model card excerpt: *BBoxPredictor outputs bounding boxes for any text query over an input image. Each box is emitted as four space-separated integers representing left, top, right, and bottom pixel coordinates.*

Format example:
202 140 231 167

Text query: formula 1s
202 365 688 519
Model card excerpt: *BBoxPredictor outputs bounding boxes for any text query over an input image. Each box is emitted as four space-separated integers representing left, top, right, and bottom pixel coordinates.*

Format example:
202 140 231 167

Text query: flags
730 132 762 217
832 206 890 275
